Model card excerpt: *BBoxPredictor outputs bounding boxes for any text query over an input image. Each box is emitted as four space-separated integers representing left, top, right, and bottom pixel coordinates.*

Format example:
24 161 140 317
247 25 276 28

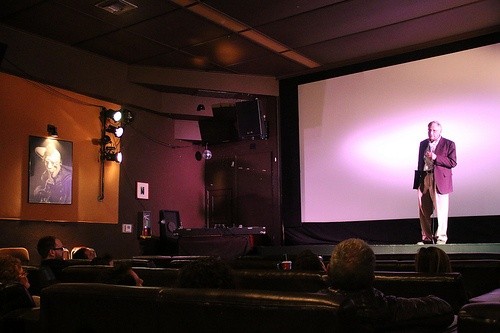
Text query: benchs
131 253 500 262
20 265 468 313
0 281 500 333
53 259 500 298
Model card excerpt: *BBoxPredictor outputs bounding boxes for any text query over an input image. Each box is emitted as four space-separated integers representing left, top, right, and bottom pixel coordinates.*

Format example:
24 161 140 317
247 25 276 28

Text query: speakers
212 107 238 142
236 99 268 140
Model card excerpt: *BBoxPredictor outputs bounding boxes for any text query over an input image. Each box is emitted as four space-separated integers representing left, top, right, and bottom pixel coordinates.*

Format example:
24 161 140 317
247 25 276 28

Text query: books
413 169 427 190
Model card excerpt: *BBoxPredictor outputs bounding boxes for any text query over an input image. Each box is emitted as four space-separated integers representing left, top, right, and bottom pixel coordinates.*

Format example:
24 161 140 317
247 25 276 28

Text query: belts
422 170 434 174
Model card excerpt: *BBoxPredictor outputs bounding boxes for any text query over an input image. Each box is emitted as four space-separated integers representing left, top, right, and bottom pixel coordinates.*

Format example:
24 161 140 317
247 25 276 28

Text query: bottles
232 224 235 228
211 224 227 229
143 218 152 236
179 221 184 229
238 224 242 228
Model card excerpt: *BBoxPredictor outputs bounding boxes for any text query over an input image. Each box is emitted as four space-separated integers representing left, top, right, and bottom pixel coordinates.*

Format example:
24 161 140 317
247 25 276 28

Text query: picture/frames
137 182 149 200
28 135 73 205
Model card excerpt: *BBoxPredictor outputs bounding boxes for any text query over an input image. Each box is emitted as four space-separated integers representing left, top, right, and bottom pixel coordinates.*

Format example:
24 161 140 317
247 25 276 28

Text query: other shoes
417 239 434 245
436 237 447 244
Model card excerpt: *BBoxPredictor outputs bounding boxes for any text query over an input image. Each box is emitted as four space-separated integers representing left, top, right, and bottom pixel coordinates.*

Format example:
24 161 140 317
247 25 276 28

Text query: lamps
107 108 122 122
105 151 123 163
106 124 124 138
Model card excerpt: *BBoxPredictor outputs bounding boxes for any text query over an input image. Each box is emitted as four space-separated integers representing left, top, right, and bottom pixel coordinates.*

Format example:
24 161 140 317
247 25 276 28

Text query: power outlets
122 224 132 233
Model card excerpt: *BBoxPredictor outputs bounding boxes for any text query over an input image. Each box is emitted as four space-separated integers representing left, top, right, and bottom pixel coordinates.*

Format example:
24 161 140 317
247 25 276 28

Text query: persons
33 146 72 204
0 235 454 333
417 120 458 244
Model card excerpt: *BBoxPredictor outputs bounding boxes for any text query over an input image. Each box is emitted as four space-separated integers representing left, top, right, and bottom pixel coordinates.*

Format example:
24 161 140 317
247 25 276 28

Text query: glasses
51 246 64 251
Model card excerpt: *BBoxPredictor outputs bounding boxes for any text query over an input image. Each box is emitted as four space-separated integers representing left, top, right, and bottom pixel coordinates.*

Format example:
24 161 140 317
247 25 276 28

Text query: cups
282 261 292 270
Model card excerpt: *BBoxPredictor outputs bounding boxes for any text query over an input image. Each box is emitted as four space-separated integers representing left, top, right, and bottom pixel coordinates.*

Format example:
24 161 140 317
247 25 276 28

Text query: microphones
429 143 432 153
